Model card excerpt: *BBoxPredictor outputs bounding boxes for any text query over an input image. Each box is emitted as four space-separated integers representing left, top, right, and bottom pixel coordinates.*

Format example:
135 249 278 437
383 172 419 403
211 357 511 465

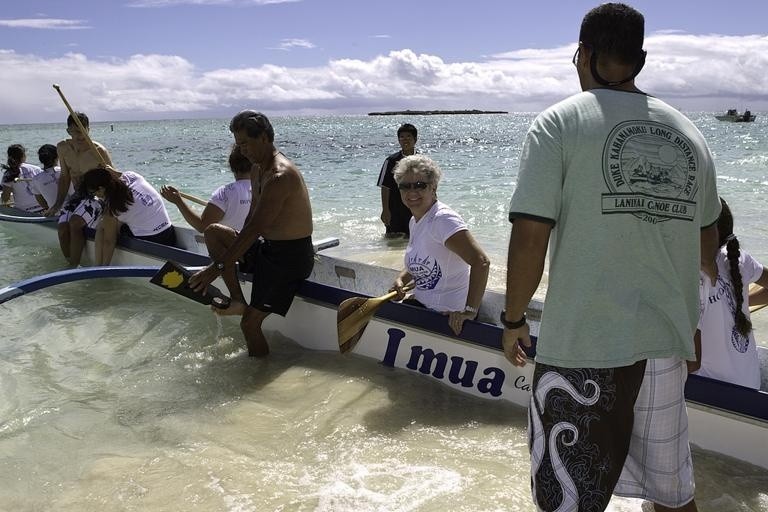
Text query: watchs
464 305 478 313
214 262 226 272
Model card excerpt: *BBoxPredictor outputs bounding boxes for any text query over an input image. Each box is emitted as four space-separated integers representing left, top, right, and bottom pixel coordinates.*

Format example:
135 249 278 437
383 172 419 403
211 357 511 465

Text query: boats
79 225 768 475
1 195 63 225
715 106 756 124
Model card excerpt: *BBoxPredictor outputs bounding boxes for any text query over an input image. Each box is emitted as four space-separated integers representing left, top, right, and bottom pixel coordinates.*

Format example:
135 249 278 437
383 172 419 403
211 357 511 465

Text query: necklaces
259 151 285 194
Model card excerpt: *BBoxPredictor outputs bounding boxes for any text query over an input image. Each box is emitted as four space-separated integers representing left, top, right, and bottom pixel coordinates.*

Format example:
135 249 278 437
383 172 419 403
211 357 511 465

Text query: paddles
749 284 767 313
337 282 416 354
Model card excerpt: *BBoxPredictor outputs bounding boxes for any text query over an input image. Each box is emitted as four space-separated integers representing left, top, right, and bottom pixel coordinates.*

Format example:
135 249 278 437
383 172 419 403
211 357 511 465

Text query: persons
377 123 417 238
686 196 768 392
500 2 722 512
188 109 314 357
0 144 43 215
32 144 76 217
42 112 114 269
81 165 176 265
386 154 490 338
159 143 253 234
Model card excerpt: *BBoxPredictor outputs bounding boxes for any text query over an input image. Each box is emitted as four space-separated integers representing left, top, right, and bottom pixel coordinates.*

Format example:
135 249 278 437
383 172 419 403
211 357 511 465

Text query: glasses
571 46 581 66
398 181 428 190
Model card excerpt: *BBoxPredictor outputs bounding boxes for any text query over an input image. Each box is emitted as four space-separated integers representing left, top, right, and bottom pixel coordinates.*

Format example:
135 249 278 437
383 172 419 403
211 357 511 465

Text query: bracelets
501 310 527 329
